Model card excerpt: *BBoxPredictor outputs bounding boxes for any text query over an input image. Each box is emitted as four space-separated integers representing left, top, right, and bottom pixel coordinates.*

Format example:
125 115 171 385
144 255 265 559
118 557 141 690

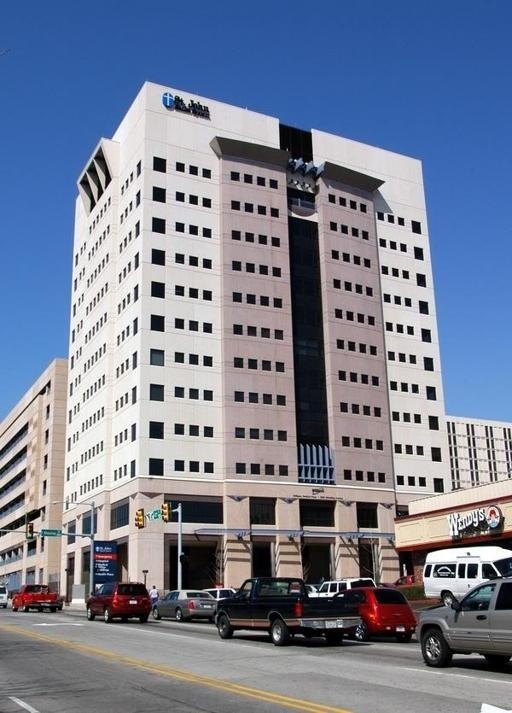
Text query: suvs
86 581 153 624
307 577 377 598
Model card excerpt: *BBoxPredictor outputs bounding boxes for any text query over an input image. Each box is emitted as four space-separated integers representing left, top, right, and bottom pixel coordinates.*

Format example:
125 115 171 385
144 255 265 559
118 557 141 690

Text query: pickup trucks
11 584 60 612
213 576 361 646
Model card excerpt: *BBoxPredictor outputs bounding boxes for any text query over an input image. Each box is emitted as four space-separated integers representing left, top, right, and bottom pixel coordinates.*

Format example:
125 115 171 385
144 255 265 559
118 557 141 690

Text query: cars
305 584 323 593
151 589 218 622
376 574 417 590
416 578 512 668
332 586 417 643
201 587 243 603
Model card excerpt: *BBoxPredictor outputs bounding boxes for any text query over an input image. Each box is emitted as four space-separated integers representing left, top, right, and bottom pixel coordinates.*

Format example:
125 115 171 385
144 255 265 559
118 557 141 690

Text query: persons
149 586 159 604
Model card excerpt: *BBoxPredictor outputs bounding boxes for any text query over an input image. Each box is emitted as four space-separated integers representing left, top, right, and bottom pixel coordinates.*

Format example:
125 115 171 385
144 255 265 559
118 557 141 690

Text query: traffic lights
161 502 173 522
26 521 33 539
135 508 146 529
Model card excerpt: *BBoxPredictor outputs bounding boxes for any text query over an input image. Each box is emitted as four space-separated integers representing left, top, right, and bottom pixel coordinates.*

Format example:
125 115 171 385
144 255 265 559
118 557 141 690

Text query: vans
420 545 512 608
0 585 9 608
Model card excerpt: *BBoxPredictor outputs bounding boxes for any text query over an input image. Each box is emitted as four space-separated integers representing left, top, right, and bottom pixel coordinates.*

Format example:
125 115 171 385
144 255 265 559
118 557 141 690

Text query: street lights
51 500 95 600
